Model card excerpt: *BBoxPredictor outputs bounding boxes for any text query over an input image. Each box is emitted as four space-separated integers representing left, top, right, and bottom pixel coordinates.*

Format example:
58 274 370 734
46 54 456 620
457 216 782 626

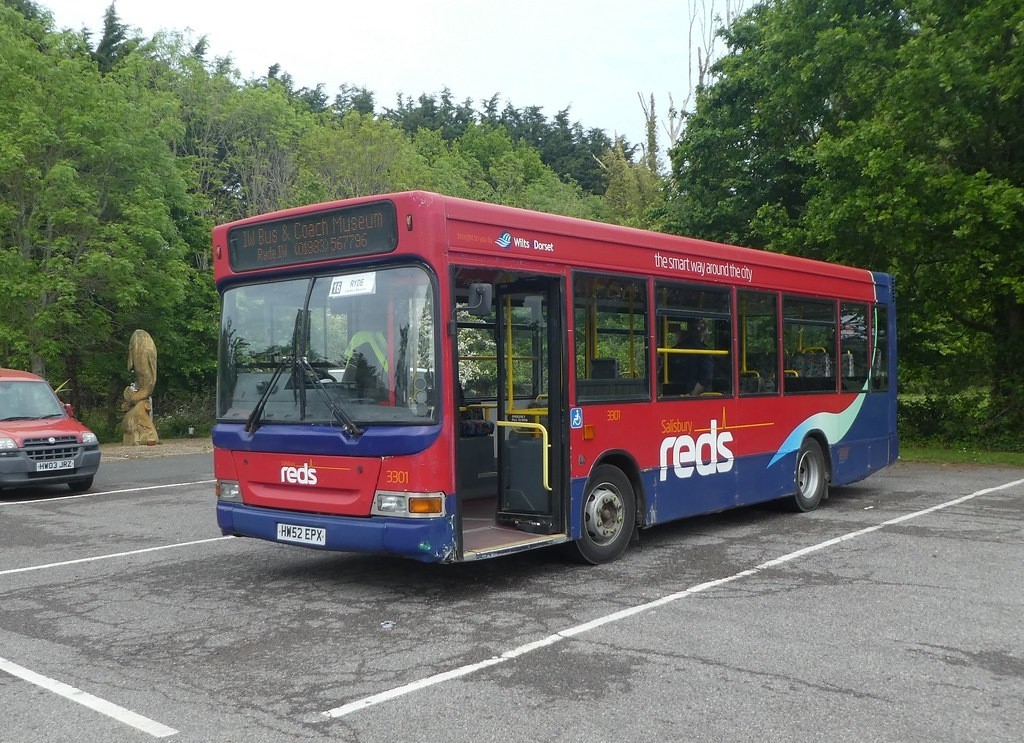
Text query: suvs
0 368 101 496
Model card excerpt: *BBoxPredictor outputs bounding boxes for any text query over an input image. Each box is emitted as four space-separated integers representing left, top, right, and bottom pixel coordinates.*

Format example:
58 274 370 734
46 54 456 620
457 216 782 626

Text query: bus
207 189 895 571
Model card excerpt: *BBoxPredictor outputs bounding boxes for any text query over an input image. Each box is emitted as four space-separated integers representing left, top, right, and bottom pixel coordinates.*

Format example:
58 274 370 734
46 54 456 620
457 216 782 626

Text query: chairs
339 331 397 398
460 346 882 439
